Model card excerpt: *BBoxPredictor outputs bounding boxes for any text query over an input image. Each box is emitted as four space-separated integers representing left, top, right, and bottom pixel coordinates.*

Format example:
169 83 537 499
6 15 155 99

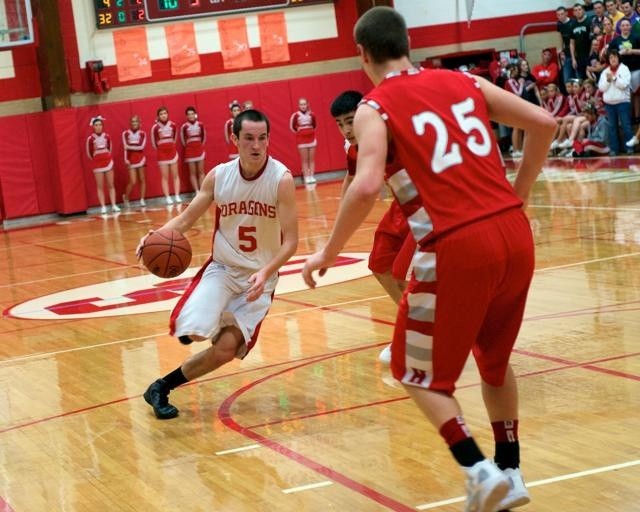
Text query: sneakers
509 138 576 157
144 379 178 419
461 459 531 512
101 194 182 214
304 176 317 184
626 136 640 147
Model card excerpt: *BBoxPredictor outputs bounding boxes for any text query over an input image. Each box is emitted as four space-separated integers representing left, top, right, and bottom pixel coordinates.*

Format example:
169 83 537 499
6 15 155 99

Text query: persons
150 108 184 205
330 89 417 362
301 6 557 511
135 109 298 419
86 114 125 213
457 0 640 161
121 115 147 208
289 98 318 184
245 100 255 109
222 101 243 157
180 105 207 194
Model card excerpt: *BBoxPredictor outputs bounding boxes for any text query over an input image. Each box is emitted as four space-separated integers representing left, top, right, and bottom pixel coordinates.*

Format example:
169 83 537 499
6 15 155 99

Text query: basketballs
142 228 192 278
